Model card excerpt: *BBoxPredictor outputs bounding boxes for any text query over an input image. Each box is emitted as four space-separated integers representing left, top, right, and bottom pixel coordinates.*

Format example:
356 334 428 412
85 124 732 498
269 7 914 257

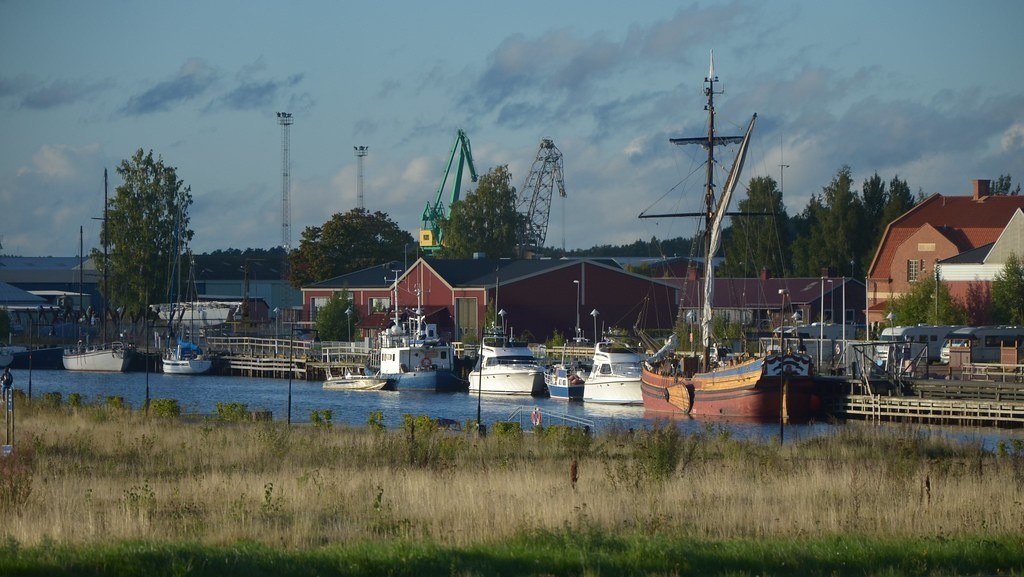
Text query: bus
940 326 1024 365
879 324 969 365
772 323 874 340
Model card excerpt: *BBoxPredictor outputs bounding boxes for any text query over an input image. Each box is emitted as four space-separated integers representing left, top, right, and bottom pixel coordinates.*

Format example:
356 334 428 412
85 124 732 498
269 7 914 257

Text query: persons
0 367 14 402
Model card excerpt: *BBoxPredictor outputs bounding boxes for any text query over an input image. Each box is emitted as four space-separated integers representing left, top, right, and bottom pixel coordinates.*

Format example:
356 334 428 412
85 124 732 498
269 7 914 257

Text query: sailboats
640 110 823 423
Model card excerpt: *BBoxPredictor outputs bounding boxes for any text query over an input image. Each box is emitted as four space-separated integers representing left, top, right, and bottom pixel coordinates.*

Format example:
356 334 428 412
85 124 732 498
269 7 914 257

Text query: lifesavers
532 407 541 426
421 358 431 367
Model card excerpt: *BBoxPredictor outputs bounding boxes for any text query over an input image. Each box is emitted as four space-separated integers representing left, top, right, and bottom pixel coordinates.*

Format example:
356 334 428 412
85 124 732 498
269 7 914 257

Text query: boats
62 340 141 372
322 363 452 390
162 345 211 375
468 336 546 396
543 364 591 401
364 286 458 391
582 340 650 405
